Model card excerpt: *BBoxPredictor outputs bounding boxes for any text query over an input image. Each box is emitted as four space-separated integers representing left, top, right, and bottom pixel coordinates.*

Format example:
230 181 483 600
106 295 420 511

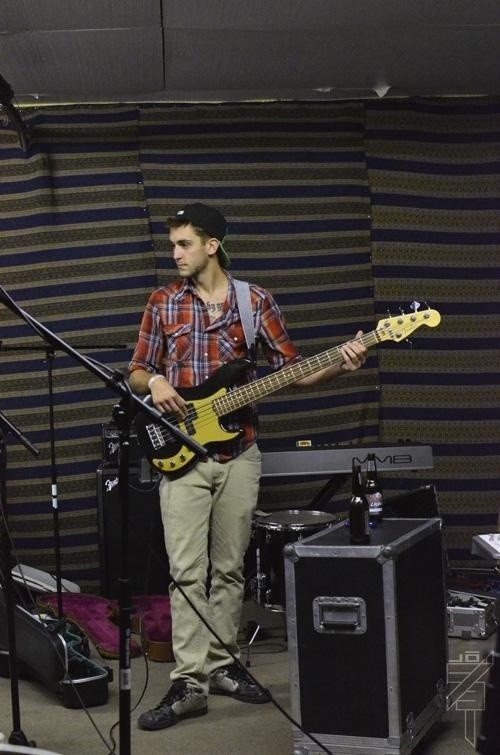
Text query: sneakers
137 681 208 729
209 664 272 704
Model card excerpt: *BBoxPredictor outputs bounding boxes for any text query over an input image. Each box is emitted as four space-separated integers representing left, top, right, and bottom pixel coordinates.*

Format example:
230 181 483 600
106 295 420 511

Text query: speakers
95 464 168 600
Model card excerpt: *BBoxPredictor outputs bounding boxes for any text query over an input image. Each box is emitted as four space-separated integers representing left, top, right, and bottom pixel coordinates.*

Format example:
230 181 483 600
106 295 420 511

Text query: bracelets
145 373 168 389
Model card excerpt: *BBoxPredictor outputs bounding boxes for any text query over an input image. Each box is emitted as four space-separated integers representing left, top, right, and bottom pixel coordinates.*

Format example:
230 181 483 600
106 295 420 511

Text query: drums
257 509 335 612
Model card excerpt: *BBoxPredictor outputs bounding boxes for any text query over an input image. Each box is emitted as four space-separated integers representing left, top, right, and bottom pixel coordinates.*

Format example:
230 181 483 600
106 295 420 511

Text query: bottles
348 452 384 545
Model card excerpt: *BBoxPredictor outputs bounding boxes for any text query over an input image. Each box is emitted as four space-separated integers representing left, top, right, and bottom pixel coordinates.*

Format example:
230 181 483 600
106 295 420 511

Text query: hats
177 202 232 270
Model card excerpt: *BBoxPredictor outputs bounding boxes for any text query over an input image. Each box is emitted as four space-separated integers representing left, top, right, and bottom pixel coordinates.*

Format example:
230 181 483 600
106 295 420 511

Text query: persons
128 205 370 732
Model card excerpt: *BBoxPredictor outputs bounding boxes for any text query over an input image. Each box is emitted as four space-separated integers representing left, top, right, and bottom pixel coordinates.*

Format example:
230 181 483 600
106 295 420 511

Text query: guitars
138 301 435 473
